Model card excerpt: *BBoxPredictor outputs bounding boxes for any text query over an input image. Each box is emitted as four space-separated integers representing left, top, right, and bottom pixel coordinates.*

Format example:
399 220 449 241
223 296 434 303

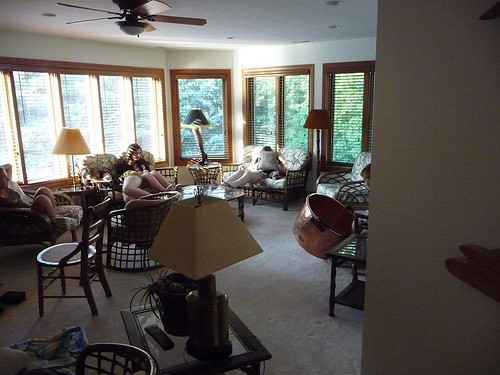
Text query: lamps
51 127 91 190
303 107 331 177
181 108 211 166
118 23 144 36
146 194 264 362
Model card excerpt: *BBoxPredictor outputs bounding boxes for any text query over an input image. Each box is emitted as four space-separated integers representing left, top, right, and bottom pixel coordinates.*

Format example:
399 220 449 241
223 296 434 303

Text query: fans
56 0 208 35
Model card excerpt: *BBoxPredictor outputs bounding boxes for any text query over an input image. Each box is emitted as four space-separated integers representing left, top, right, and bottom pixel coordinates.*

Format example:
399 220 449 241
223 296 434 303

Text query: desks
187 161 221 185
323 232 368 319
120 292 272 375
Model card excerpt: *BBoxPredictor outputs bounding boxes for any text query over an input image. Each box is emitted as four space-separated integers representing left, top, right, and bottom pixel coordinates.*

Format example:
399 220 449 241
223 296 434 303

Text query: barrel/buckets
294 193 360 260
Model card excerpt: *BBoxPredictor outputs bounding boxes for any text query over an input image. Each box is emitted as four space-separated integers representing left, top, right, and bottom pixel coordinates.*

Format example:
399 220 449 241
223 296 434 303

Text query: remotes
145 323 174 351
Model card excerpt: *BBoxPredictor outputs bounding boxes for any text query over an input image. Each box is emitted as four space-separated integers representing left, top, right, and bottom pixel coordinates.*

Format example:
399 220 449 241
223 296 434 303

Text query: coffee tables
176 184 246 223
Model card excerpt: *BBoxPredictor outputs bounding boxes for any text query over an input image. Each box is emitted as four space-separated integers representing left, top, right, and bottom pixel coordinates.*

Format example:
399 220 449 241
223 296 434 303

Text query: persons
336 163 370 266
211 146 288 187
0 166 79 220
88 167 162 204
119 143 182 192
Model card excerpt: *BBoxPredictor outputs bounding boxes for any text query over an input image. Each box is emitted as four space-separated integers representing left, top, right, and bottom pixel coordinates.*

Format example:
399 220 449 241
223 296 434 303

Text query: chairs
315 151 370 235
0 165 177 317
4 326 158 375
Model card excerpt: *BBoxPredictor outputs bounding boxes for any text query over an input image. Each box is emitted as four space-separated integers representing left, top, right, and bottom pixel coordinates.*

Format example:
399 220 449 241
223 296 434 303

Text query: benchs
220 145 311 211
83 150 155 200
